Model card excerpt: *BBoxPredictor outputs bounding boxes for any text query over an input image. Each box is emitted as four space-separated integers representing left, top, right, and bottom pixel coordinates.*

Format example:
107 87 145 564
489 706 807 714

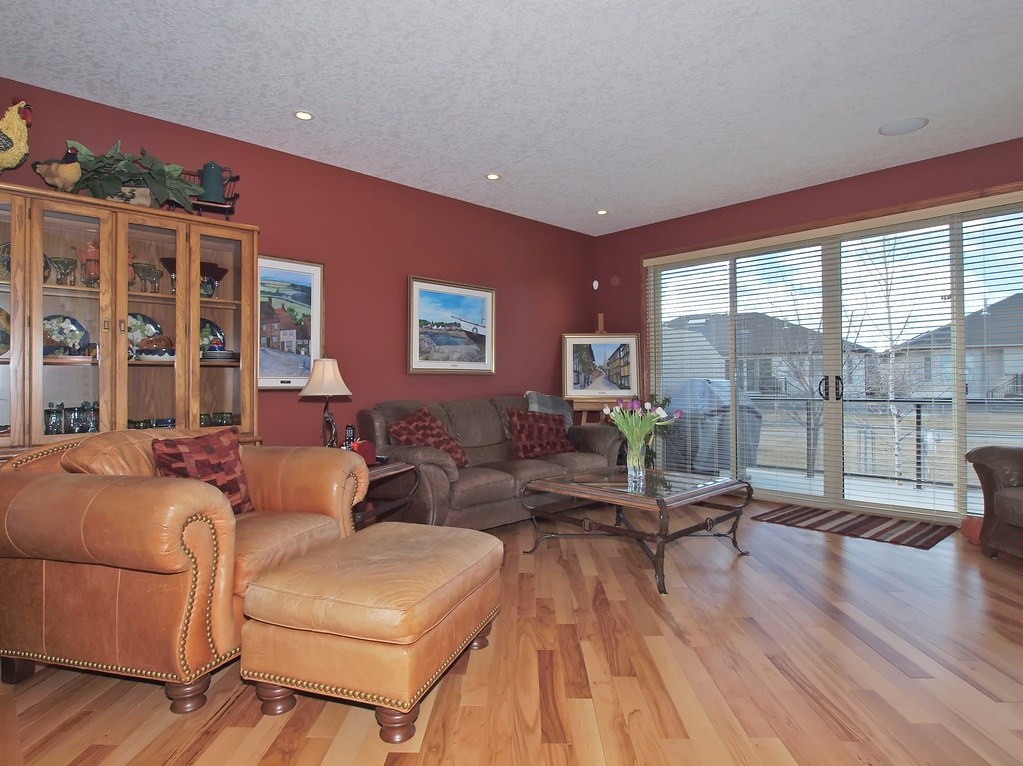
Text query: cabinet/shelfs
0 182 261 460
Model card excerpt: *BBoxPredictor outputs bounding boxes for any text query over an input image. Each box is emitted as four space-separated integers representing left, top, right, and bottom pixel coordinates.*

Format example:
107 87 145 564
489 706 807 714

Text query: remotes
345 424 356 452
376 455 388 463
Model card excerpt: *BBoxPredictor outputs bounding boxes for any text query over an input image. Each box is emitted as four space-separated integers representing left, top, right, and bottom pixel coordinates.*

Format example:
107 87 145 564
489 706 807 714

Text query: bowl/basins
154 419 175 427
134 348 176 361
203 350 233 358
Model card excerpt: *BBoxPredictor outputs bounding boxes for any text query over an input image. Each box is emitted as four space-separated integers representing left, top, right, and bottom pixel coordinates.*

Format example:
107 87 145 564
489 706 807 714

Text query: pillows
385 403 468 473
506 405 578 460
148 426 256 513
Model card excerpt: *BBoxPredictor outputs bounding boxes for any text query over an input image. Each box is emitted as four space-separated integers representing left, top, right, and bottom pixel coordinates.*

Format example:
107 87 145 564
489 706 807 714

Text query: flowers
601 390 684 464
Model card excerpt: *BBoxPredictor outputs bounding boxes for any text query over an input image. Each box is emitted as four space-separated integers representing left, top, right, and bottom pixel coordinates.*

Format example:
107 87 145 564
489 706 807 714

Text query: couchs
964 445 1023 559
359 395 624 530
0 425 373 717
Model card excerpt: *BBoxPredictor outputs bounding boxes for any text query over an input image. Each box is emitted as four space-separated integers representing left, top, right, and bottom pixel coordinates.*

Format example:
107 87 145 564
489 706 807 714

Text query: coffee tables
518 463 754 595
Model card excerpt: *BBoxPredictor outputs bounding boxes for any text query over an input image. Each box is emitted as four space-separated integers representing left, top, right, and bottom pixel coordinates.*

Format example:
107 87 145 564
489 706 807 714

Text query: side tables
355 457 420 525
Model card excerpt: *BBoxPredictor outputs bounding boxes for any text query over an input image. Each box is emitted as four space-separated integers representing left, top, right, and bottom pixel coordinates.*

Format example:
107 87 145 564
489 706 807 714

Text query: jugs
196 160 231 205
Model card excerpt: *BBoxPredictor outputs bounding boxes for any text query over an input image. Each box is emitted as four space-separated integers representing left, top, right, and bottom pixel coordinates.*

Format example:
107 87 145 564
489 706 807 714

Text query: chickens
0 96 33 175
31 147 81 194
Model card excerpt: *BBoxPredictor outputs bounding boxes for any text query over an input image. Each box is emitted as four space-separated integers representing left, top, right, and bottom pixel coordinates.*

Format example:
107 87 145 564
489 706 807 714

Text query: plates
0 308 13 357
200 358 238 361
41 314 90 356
125 312 163 357
199 318 225 352
0 240 51 284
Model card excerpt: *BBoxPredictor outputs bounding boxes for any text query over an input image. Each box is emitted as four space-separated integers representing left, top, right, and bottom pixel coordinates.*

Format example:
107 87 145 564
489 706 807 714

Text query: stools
237 519 507 746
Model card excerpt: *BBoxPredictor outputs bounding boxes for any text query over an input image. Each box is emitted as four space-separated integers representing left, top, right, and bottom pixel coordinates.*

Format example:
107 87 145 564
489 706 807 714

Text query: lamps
297 354 353 451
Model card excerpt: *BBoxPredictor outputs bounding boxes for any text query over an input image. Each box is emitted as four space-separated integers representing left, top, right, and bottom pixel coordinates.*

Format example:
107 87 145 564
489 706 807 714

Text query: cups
43 401 101 434
199 411 236 428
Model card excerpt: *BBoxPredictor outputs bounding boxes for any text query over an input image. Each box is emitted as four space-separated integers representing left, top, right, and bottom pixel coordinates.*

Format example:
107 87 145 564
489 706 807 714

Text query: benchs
164 163 240 221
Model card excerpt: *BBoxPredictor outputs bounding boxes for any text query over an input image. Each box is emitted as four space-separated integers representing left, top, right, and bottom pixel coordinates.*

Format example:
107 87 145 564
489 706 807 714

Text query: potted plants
65 139 206 215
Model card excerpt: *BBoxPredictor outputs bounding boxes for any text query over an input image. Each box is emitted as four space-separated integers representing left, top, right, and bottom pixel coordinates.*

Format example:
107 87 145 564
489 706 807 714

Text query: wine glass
49 254 102 288
134 258 229 298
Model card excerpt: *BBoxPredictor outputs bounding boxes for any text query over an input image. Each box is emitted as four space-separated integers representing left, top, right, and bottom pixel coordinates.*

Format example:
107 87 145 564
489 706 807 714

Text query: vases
626 439 649 495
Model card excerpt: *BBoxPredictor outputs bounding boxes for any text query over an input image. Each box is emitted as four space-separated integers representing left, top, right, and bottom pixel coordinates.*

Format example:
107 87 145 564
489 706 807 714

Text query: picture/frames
561 332 642 401
258 254 327 391
406 272 499 377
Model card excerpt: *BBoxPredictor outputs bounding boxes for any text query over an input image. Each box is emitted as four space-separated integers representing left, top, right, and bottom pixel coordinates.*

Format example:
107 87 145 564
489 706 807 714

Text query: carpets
750 503 958 552
